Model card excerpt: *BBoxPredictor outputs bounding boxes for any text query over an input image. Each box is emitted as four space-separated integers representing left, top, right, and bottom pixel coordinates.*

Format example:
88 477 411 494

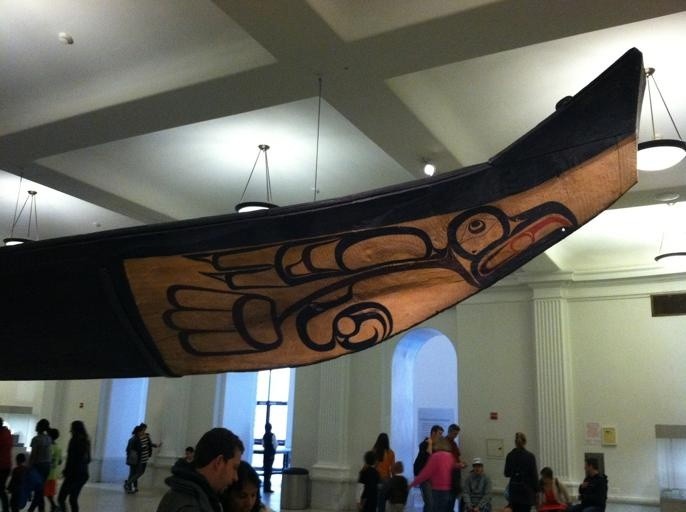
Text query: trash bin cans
282 468 308 509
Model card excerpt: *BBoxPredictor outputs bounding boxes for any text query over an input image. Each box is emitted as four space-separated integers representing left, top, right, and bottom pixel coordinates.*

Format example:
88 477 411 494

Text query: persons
174 447 196 471
124 426 144 494
223 460 272 512
0 418 91 512
358 424 608 511
132 423 162 493
156 428 244 511
262 423 278 492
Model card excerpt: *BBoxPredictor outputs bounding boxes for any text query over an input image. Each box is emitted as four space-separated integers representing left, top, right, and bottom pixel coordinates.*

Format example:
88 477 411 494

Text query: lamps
655 201 686 269
235 144 281 213
3 190 39 246
636 67 686 172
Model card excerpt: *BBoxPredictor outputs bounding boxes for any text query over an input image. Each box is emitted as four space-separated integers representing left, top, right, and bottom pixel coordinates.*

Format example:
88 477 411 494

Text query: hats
471 457 484 466
